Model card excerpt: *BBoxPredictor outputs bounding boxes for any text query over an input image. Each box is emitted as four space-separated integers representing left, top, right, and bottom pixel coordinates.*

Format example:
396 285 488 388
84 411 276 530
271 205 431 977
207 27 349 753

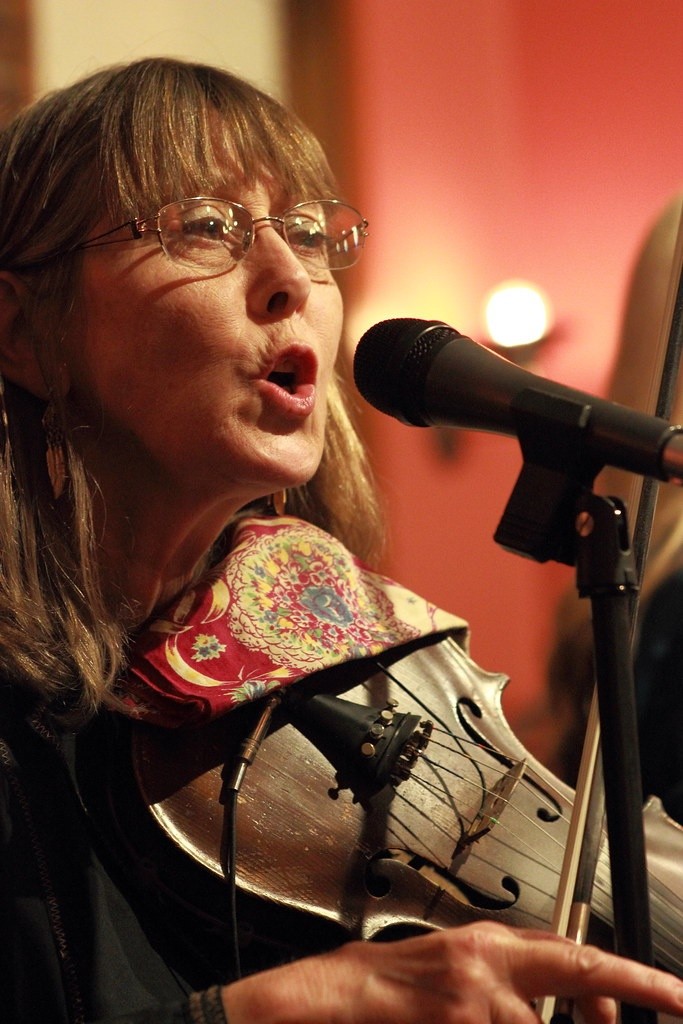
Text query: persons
0 57 682 1024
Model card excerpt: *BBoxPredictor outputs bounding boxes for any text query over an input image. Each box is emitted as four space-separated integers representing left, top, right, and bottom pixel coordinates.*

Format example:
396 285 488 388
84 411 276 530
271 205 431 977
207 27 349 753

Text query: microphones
353 318 683 487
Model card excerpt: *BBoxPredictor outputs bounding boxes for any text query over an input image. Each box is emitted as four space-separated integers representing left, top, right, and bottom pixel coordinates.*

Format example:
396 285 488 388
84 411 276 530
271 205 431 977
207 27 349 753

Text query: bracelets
192 986 227 1024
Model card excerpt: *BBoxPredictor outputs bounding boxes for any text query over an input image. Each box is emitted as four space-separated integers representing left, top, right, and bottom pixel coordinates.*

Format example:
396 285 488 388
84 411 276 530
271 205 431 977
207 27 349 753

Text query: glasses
74 196 369 270
